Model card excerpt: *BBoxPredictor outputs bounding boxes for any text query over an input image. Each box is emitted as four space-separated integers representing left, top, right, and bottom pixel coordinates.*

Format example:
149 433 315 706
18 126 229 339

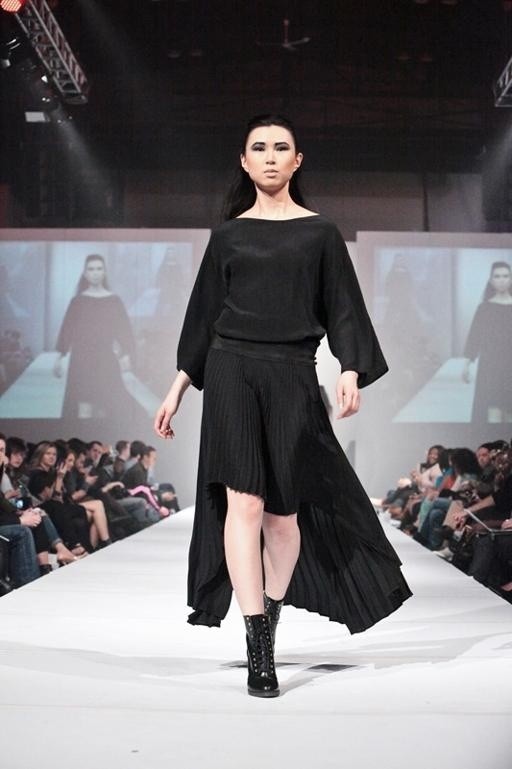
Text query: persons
48 252 148 421
458 258 512 425
373 438 511 604
0 435 185 598
155 113 412 698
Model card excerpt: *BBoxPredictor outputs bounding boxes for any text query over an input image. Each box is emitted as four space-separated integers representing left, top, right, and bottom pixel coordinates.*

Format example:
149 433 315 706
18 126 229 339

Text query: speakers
243 592 285 697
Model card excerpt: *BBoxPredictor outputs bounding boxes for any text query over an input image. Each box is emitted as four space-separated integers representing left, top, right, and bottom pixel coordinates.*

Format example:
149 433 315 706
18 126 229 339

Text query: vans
57 552 87 565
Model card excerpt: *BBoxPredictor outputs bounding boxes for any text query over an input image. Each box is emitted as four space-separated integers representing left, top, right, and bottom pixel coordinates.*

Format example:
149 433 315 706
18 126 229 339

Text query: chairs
433 547 455 561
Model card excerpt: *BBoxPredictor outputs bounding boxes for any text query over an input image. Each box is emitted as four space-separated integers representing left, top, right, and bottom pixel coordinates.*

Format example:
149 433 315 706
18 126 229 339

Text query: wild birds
464 506 511 538
